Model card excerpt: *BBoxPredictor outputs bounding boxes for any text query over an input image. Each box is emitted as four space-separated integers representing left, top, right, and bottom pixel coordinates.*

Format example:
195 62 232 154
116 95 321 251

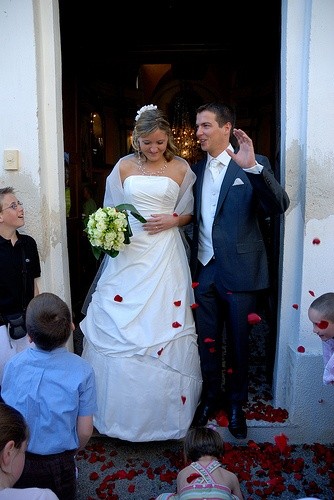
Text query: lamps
165 102 200 164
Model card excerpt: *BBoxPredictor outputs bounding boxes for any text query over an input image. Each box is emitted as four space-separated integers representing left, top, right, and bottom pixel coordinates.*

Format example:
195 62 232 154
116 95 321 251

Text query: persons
156 429 243 499
81 185 97 228
101 104 202 443
0 401 58 499
0 293 96 500
189 102 290 438
0 187 41 327
308 293 334 386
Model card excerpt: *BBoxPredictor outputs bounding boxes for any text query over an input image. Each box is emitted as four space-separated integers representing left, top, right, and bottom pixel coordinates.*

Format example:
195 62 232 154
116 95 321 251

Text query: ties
207 160 221 182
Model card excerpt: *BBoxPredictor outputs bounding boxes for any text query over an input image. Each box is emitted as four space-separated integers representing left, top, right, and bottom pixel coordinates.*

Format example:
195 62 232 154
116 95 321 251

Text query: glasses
1 201 23 214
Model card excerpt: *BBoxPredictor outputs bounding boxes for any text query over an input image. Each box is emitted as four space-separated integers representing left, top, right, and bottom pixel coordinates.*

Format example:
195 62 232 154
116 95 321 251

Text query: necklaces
136 157 167 176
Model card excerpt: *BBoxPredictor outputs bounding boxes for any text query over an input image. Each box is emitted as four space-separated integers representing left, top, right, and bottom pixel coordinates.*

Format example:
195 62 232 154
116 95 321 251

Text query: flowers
83 204 147 260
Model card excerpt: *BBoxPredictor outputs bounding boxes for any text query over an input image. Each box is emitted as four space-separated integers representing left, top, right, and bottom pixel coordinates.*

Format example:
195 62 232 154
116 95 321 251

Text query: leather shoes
224 401 249 440
188 398 221 432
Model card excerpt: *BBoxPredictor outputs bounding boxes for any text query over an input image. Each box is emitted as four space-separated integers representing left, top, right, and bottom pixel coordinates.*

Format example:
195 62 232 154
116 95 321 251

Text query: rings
154 225 158 229
